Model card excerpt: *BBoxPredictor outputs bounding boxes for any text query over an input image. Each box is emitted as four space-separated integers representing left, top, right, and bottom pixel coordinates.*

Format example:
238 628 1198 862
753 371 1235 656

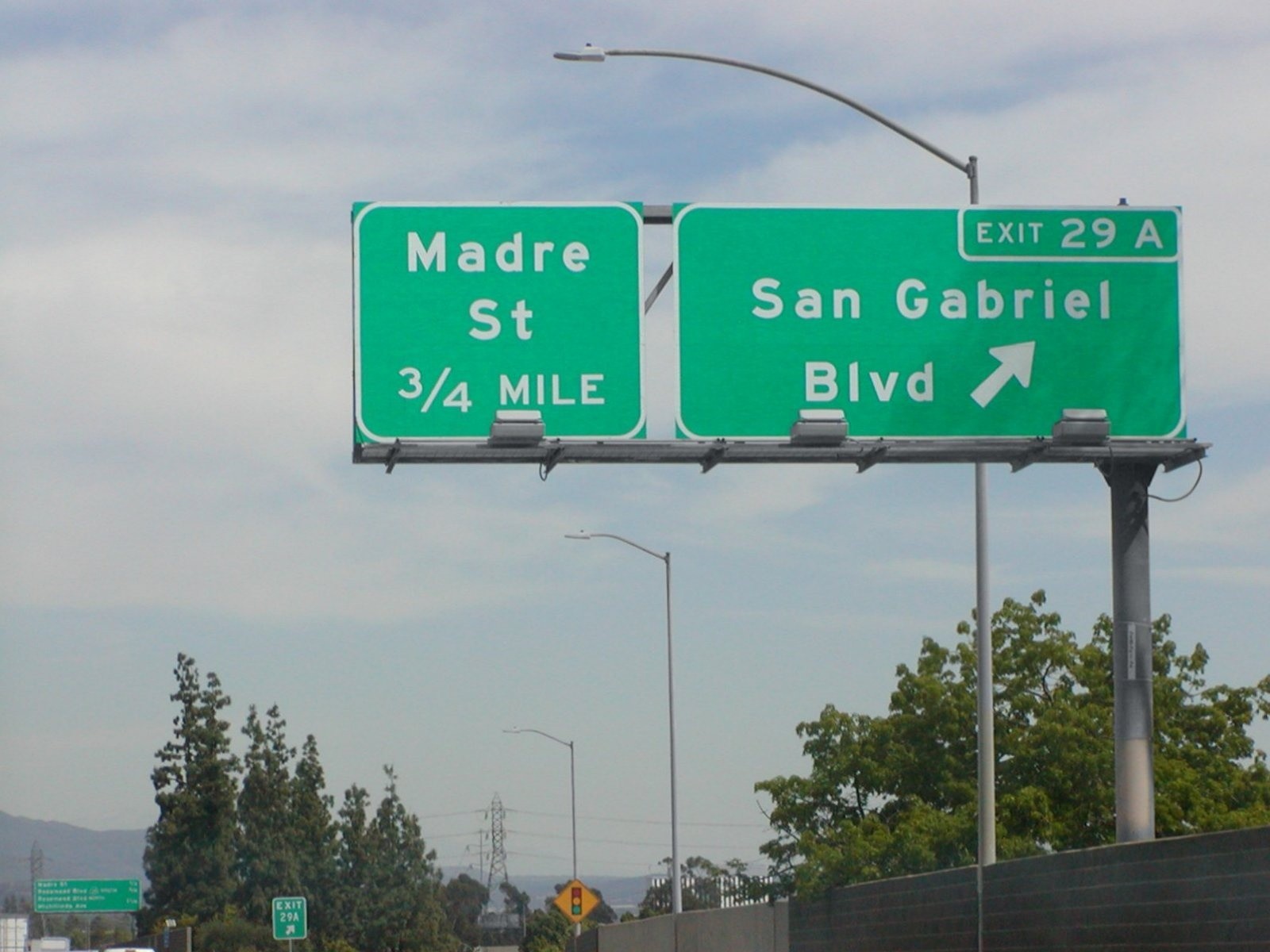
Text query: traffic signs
350 202 645 438
272 897 308 942
32 878 140 913
673 200 1188 444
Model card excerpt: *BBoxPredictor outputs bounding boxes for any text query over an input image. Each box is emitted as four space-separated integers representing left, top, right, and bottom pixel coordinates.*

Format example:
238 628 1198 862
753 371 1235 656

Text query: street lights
566 528 680 914
556 46 997 867
501 726 584 952
